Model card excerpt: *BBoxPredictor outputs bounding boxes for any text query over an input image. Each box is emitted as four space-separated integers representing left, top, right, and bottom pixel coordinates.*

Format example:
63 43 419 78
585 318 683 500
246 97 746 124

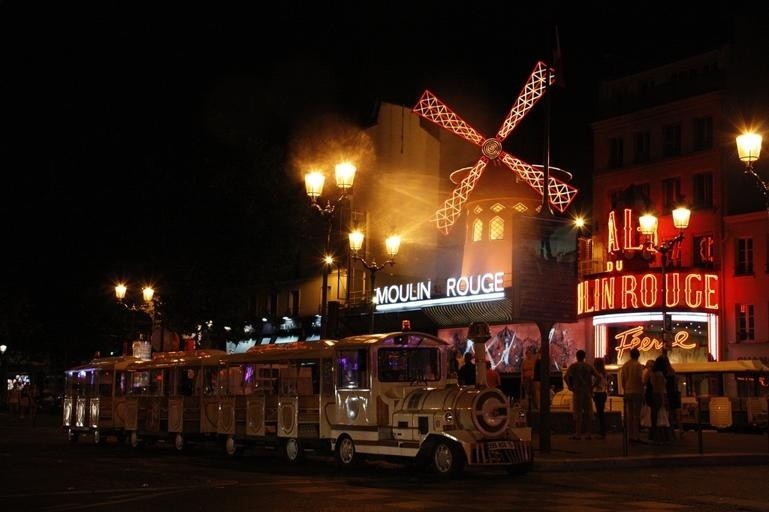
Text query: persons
485 361 501 388
519 346 683 445
457 352 476 386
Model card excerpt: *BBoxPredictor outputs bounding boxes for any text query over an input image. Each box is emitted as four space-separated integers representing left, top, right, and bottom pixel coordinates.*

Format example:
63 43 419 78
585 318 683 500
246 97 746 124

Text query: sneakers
628 427 680 444
568 433 608 440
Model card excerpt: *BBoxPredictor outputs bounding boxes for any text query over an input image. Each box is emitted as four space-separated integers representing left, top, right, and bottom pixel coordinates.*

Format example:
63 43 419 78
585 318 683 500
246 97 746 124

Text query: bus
673 360 768 430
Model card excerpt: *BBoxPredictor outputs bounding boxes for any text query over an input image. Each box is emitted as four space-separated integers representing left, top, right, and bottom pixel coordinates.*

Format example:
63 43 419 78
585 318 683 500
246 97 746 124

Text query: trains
64 327 536 483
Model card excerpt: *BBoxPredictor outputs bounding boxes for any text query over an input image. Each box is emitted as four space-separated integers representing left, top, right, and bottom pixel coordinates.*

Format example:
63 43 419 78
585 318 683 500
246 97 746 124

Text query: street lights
111 281 164 347
347 230 401 325
640 206 692 354
305 161 356 339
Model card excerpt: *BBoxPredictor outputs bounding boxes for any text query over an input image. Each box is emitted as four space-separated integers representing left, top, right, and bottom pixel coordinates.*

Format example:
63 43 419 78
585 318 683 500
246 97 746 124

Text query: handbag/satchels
639 398 652 428
645 377 654 407
655 407 671 428
